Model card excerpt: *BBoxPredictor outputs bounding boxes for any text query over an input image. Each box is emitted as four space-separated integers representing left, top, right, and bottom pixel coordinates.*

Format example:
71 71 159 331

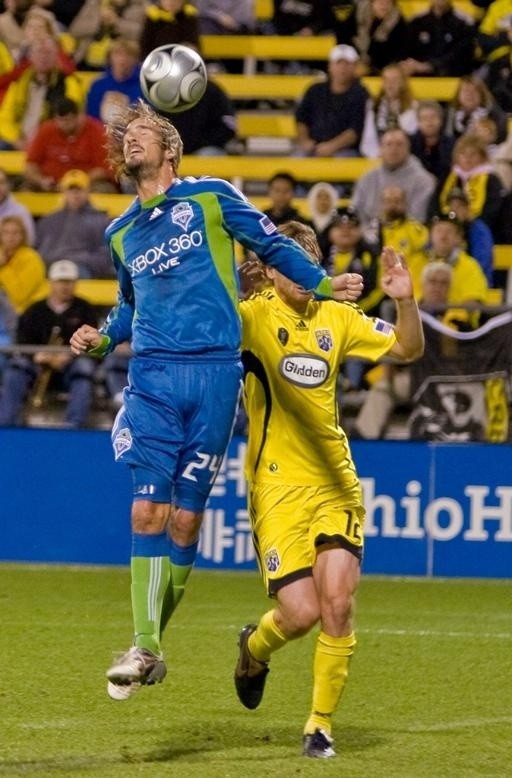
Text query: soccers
139 44 208 113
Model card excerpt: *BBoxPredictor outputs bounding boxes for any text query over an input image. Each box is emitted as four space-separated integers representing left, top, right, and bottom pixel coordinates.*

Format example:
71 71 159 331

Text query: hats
328 43 360 65
49 259 80 281
59 168 90 191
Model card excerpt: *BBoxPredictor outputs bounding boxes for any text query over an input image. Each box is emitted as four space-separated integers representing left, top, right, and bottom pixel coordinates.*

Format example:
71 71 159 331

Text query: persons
69 103 364 700
0 1 512 437
238 219 426 760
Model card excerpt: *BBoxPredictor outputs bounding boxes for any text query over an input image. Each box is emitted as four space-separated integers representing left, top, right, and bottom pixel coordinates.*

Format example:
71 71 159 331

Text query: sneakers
302 729 336 760
106 645 168 687
106 679 138 701
233 623 269 710
